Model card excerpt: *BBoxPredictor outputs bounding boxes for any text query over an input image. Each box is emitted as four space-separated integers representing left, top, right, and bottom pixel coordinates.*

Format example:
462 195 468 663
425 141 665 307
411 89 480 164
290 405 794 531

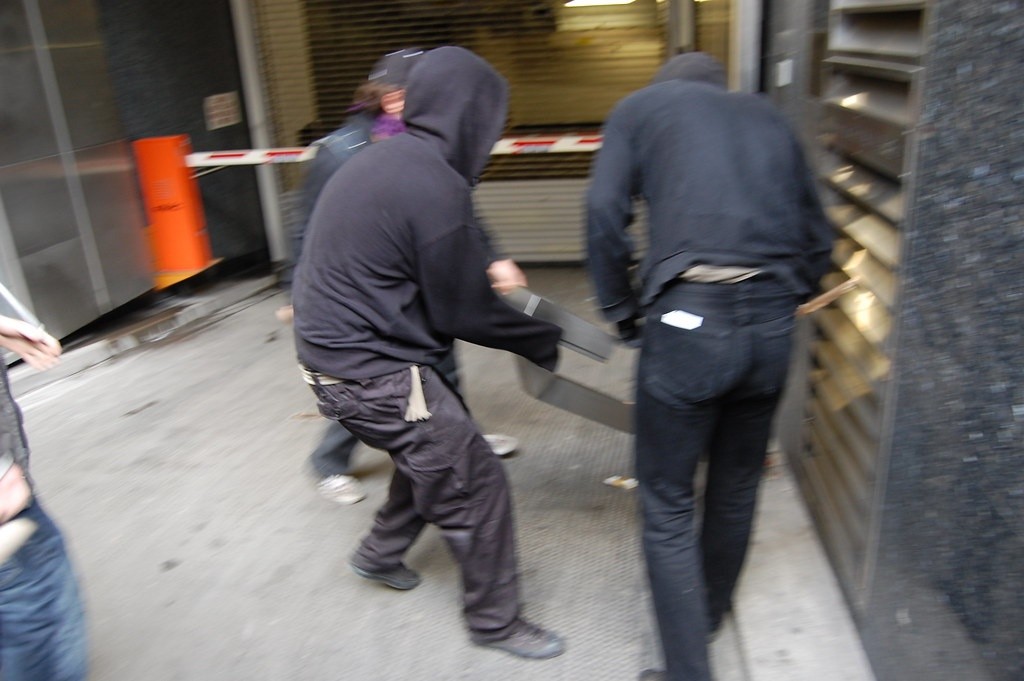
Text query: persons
0 312 91 681
581 52 835 681
277 44 566 661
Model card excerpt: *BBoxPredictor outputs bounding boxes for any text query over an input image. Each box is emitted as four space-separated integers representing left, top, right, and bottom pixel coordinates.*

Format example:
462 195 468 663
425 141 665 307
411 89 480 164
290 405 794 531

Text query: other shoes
474 619 567 660
482 432 518 456
350 560 419 590
320 472 367 505
638 668 665 681
705 616 721 643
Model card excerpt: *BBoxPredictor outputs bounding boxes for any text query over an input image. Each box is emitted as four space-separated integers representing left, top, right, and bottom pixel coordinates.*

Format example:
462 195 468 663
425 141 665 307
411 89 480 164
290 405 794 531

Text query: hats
367 49 424 89
